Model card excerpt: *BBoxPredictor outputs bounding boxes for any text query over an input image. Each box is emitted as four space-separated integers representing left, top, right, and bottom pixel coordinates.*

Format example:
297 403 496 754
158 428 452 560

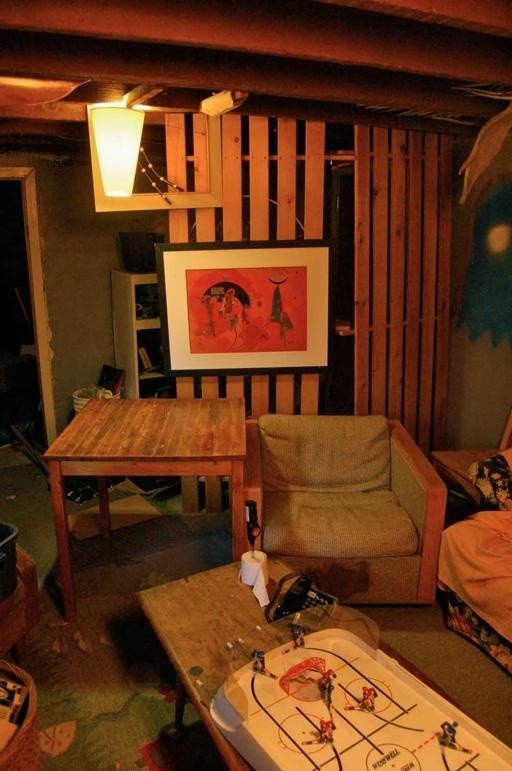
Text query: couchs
436 447 512 676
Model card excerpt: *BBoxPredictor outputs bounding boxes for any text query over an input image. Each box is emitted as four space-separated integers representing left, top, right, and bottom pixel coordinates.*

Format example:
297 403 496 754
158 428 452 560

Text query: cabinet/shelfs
111 268 165 398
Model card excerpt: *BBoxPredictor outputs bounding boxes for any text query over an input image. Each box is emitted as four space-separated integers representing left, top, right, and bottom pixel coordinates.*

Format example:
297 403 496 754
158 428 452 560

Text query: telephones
265 572 339 633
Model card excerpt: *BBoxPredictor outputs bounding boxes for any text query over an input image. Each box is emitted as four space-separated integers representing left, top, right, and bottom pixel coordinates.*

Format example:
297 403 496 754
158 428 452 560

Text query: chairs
431 406 512 511
243 414 448 605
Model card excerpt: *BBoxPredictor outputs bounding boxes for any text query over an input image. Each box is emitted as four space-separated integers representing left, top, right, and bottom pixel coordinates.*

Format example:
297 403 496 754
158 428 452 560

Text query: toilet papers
240 549 270 607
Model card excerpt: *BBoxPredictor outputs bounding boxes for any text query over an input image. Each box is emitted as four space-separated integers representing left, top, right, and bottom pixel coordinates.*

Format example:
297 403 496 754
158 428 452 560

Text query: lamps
91 106 145 198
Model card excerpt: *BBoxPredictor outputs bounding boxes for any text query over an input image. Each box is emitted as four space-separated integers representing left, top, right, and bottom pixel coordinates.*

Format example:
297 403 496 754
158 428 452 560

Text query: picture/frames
154 239 336 377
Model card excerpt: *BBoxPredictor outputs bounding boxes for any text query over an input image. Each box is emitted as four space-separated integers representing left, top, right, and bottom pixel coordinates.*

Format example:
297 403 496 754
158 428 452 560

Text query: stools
0 545 40 667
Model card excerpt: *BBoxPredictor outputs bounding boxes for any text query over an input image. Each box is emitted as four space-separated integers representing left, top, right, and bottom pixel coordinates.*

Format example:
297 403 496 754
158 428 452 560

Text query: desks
43 398 246 623
135 555 512 771
